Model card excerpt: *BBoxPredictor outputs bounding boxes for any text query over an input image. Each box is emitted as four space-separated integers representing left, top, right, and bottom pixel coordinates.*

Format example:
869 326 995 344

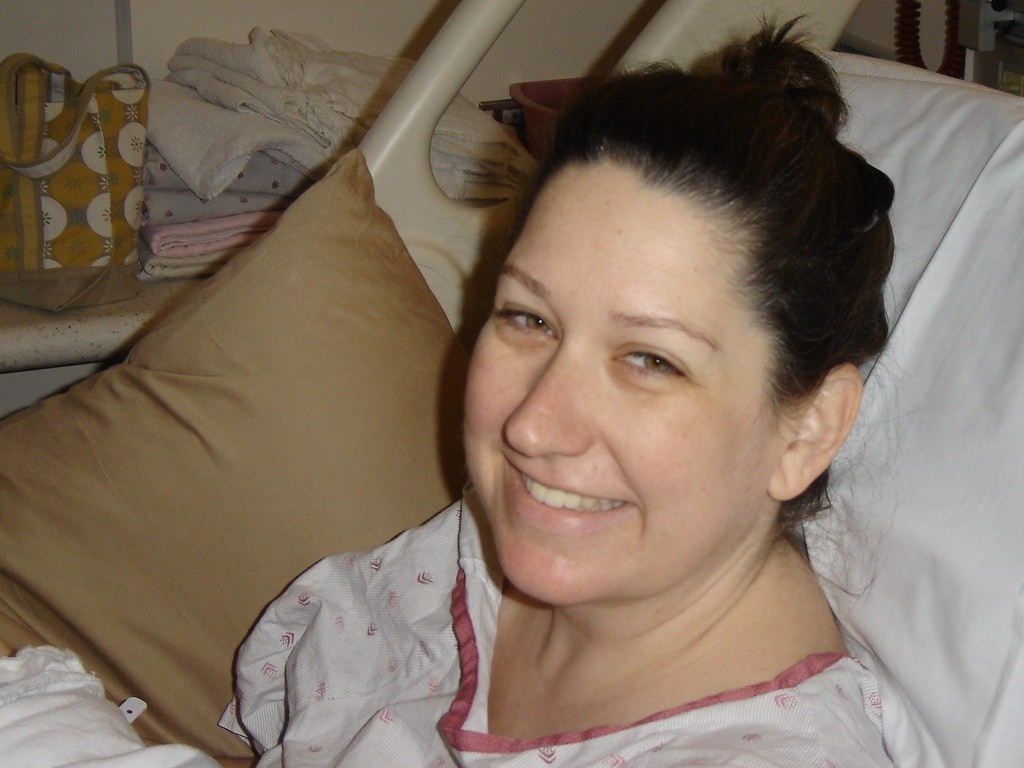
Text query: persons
223 14 948 767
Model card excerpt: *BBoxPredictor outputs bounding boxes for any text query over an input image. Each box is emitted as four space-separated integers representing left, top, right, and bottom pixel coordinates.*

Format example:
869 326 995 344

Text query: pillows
0 149 470 764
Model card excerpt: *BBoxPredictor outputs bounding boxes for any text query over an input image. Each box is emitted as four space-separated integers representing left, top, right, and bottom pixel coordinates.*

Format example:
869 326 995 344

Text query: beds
0 1 1023 766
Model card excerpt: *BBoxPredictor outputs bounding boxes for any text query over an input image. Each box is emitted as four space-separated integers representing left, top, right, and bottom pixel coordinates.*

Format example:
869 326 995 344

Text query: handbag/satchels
0 52 152 311
